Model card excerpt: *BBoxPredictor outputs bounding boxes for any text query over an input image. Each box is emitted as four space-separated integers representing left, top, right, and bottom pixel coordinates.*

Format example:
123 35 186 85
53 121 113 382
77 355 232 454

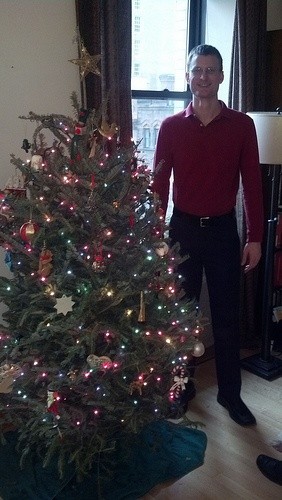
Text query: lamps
240 107 282 382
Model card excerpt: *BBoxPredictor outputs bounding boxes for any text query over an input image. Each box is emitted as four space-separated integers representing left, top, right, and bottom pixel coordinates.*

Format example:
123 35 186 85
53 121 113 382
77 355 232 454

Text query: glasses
189 67 221 74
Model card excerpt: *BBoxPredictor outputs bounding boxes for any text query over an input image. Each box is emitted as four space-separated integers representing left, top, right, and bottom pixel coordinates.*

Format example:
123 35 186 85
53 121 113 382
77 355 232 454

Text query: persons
149 44 264 426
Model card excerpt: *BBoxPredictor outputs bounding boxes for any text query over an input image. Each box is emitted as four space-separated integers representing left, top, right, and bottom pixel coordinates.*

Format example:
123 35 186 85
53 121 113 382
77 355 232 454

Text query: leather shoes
171 382 195 419
257 454 282 485
217 391 256 427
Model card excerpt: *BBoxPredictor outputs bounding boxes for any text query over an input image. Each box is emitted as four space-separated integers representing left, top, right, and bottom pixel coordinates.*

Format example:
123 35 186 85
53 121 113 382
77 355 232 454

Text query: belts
173 208 235 228
